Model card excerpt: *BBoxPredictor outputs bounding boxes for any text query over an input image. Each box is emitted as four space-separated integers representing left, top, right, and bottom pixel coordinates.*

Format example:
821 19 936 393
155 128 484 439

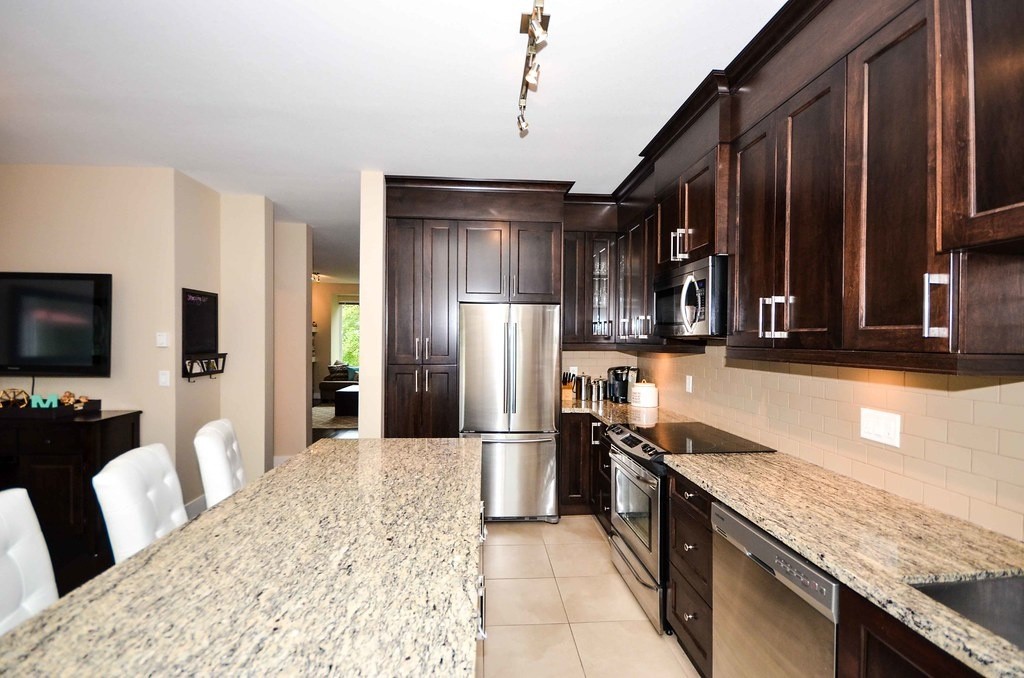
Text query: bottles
630 406 658 430
631 383 659 407
312 322 317 327
575 375 607 402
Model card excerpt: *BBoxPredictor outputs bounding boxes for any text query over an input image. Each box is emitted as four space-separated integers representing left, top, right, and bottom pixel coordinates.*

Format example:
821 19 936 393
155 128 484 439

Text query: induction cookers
605 422 777 464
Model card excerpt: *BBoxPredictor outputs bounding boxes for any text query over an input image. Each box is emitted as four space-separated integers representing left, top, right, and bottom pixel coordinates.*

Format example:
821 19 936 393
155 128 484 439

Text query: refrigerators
458 302 559 524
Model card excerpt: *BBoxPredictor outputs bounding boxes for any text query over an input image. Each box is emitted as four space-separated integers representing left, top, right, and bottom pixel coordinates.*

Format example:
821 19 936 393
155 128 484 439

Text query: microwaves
654 255 727 339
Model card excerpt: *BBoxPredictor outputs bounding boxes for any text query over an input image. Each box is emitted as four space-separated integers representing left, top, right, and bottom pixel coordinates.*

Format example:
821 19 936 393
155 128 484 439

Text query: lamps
516 0 551 130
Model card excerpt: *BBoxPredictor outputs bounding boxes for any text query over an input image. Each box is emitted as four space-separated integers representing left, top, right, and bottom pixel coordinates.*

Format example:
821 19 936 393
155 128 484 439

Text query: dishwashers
710 502 839 678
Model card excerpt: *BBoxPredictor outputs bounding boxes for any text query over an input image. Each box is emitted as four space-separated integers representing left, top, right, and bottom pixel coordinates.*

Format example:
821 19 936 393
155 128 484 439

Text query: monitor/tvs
0 271 112 379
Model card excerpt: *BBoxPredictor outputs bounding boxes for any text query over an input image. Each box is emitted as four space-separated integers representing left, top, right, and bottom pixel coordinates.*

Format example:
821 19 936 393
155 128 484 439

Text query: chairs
0 486 60 636
193 418 247 509
92 438 191 566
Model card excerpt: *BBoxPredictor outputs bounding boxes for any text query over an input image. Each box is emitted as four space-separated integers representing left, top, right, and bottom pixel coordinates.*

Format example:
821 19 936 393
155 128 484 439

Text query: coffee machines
607 365 640 403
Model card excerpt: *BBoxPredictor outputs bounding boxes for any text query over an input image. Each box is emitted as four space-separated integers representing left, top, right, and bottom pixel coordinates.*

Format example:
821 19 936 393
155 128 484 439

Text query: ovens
608 444 673 637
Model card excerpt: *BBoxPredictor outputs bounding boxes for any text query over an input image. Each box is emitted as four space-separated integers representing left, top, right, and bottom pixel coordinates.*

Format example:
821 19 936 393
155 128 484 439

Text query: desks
336 383 359 415
0 437 483 678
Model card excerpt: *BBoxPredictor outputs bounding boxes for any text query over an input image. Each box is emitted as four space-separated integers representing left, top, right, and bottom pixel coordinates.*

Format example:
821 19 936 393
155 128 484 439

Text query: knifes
562 371 575 385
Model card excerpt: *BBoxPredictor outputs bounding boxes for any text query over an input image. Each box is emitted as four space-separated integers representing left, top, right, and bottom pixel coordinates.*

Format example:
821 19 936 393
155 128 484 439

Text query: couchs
319 360 359 402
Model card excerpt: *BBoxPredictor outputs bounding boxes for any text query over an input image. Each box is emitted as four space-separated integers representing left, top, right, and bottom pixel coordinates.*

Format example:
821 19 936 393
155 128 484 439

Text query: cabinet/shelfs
837 588 987 678
386 218 458 437
726 56 845 350
618 202 658 344
458 220 562 304
659 145 714 265
940 0 1024 249
0 411 142 572
844 0 956 353
663 476 714 678
560 413 612 534
562 229 617 344
